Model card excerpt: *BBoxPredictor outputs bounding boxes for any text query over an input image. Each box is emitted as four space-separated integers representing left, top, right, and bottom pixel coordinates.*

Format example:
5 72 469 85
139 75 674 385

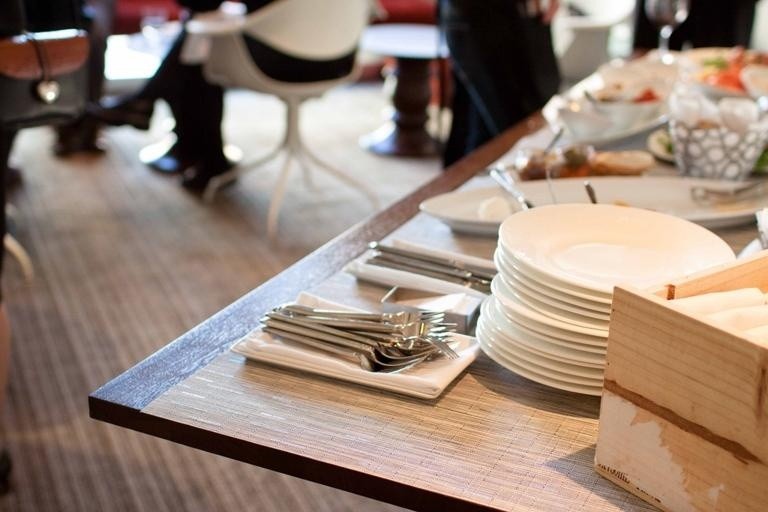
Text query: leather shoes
85 100 154 127
146 140 198 172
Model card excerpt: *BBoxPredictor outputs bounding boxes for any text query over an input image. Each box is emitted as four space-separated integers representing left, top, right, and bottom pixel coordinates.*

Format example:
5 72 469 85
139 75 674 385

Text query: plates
474 201 738 398
419 176 768 239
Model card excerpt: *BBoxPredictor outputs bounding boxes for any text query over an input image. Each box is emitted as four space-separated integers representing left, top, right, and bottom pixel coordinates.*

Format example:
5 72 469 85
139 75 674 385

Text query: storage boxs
592 249 767 512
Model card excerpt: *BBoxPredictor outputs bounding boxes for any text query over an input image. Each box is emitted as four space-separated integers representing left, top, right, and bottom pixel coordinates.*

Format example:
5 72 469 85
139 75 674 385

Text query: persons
630 0 756 59
439 1 563 170
87 1 354 190
0 0 109 188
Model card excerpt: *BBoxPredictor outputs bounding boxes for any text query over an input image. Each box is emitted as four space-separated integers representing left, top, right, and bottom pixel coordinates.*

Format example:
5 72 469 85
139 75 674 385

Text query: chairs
186 1 380 242
549 1 637 95
1 120 35 289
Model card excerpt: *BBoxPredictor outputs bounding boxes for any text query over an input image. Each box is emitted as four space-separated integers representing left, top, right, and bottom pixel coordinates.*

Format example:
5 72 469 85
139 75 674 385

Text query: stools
357 22 454 157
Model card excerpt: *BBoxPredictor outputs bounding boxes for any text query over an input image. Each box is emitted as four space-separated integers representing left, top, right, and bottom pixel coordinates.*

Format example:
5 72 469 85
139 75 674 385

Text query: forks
260 303 459 373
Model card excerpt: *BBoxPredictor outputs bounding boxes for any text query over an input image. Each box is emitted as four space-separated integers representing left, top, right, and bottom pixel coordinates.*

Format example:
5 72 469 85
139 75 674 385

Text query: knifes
364 241 497 294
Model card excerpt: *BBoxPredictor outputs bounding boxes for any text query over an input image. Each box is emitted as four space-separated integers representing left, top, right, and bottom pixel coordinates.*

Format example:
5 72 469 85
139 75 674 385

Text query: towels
234 291 481 395
354 235 496 302
